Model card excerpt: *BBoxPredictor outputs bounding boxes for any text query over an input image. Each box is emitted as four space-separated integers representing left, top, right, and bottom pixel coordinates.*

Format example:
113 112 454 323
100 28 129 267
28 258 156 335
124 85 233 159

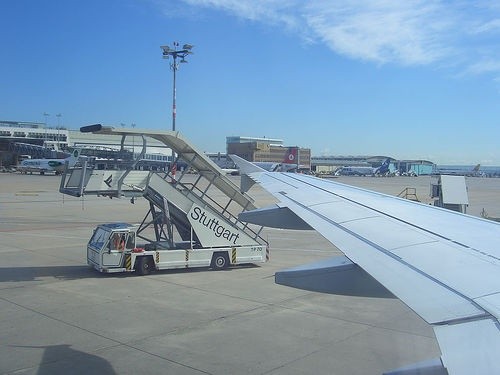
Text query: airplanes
11 140 82 176
219 146 298 176
334 157 390 179
224 146 500 375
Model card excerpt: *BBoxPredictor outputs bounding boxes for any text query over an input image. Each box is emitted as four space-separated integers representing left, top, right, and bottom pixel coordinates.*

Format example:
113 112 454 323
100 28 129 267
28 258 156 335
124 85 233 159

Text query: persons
113 234 124 253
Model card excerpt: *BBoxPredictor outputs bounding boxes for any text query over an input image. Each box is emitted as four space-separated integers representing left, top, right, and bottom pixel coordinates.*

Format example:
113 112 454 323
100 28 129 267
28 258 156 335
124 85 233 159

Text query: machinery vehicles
58 123 269 276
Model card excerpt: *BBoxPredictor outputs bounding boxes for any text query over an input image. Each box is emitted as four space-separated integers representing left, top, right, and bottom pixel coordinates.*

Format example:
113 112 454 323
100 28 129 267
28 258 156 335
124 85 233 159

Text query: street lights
160 42 195 185
